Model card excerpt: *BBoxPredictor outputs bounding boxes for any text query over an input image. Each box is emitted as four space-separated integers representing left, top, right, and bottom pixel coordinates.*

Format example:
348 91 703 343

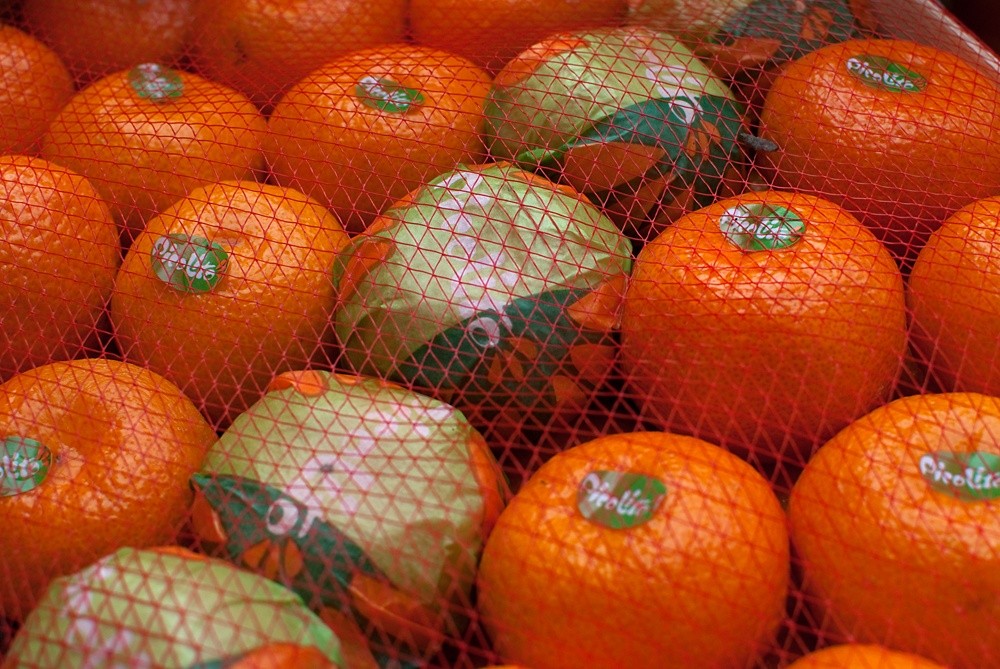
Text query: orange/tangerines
0 0 1000 669
